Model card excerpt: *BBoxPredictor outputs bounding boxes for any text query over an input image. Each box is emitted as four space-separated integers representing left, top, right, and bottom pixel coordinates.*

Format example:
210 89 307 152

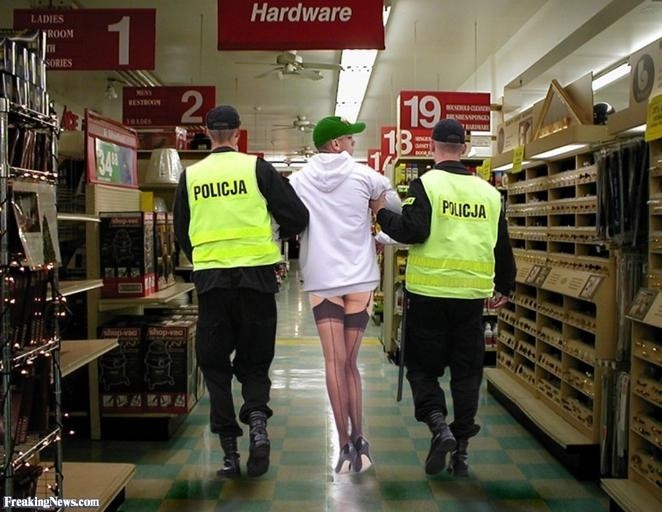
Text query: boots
423 411 457 476
215 431 242 477
244 411 272 478
447 435 469 478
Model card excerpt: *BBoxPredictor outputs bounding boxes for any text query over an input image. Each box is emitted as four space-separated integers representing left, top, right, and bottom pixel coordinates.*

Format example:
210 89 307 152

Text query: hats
431 119 466 146
312 116 365 148
206 105 242 131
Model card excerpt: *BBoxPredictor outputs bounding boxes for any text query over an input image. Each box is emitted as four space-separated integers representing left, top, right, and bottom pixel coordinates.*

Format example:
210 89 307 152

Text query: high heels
335 443 358 475
350 435 373 474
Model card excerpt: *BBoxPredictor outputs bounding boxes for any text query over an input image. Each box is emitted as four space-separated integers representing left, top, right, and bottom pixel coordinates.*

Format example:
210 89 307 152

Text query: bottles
483 294 499 350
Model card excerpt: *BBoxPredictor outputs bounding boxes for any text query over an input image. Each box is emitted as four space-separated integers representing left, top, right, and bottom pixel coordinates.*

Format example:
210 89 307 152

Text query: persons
370 120 517 475
174 106 309 480
272 116 404 474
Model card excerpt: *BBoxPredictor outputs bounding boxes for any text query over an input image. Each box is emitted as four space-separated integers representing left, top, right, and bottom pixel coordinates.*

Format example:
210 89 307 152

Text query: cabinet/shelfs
0 27 63 511
478 123 618 450
600 104 662 512
382 155 496 365
37 213 137 512
86 184 196 440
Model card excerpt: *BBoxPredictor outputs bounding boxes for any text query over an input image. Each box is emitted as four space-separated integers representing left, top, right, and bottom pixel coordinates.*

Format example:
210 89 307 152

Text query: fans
235 52 345 80
286 146 314 158
266 155 308 167
272 115 317 133
